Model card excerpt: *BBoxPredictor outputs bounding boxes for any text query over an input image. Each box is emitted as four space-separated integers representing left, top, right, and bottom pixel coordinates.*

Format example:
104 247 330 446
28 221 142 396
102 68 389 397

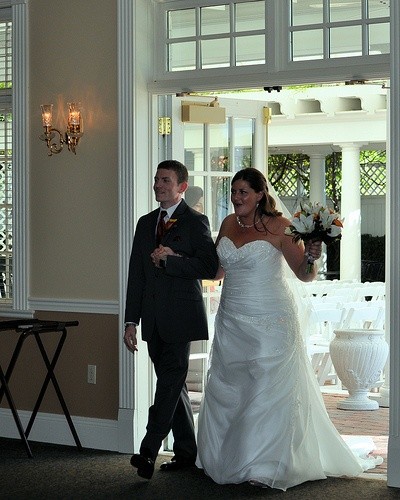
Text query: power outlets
87 365 96 384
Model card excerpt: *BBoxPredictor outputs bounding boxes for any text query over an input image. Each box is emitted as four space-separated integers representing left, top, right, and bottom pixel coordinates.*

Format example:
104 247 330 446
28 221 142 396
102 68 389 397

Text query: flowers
165 218 178 230
285 202 345 274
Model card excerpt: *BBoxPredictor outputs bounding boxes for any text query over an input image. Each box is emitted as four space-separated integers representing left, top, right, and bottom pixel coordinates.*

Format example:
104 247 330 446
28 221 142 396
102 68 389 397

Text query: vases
329 329 388 411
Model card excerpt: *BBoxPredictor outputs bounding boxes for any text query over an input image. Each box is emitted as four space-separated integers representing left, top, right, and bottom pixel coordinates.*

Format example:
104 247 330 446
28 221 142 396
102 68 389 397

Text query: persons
123 160 218 479
185 186 204 216
151 169 322 489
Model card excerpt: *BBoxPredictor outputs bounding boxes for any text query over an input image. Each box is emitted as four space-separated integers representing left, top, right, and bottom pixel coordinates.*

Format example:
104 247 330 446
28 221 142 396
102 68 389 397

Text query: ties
156 210 167 240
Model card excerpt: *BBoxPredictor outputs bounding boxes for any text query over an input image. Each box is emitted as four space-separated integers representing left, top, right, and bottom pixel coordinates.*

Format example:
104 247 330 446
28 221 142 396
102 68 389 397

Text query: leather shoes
160 456 187 470
130 454 154 480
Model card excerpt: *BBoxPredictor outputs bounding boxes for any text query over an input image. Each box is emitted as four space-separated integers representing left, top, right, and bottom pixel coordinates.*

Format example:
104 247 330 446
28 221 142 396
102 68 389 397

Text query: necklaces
237 215 263 227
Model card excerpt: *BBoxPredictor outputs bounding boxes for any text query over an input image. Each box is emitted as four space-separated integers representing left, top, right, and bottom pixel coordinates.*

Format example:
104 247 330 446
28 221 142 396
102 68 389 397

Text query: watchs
159 256 167 268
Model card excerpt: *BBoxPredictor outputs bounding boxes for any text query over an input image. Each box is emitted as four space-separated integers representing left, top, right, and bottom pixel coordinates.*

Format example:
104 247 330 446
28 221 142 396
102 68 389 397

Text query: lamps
38 102 84 158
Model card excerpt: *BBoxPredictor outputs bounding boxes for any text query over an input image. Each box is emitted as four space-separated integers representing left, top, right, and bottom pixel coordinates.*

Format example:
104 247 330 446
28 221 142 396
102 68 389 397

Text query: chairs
289 279 386 390
